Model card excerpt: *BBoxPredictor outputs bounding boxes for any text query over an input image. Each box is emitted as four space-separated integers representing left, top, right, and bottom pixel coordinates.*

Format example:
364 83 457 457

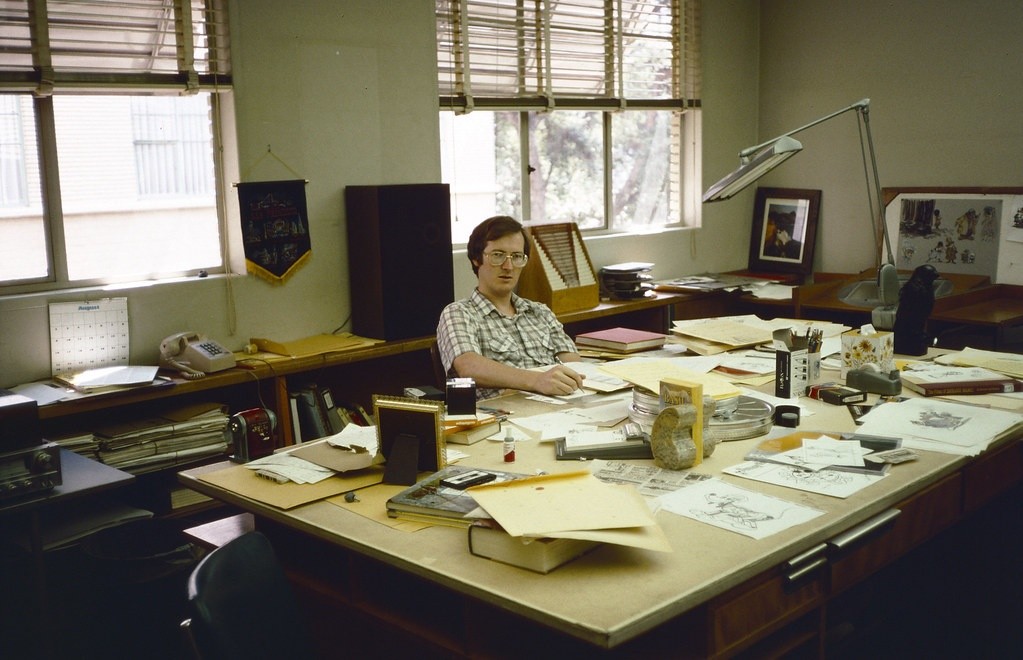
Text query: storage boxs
772 327 821 400
805 382 868 405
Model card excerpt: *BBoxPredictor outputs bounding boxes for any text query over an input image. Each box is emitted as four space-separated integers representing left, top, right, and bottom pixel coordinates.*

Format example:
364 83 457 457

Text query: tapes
857 363 881 375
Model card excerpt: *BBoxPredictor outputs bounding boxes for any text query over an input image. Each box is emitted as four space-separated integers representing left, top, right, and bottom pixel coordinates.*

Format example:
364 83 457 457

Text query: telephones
160 331 236 372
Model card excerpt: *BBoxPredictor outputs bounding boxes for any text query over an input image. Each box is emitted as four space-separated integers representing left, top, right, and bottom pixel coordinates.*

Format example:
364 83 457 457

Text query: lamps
701 99 899 309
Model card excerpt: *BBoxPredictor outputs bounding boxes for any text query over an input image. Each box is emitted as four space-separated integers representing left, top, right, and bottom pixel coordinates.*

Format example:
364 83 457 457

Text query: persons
437 216 586 401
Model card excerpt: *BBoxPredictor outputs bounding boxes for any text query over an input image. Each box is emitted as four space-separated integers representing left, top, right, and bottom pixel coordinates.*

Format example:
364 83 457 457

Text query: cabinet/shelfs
0 270 1023 550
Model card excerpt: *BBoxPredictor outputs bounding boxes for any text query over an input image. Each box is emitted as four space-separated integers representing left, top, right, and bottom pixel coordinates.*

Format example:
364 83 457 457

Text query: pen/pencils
788 328 797 336
555 356 585 394
476 405 511 416
806 327 824 353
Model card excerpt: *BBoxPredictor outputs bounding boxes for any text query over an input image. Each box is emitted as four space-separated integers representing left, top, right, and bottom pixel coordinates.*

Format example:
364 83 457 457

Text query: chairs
178 532 308 660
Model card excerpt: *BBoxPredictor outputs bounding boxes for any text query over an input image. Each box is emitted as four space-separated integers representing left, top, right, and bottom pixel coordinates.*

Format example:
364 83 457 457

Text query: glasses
482 250 529 268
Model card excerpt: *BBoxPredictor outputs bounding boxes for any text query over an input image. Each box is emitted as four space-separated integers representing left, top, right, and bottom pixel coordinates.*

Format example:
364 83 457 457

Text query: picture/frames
877 187 1023 284
373 394 446 486
748 186 822 275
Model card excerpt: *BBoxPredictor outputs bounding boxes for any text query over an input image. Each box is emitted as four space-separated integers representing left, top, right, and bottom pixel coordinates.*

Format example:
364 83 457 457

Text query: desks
178 324 1023 647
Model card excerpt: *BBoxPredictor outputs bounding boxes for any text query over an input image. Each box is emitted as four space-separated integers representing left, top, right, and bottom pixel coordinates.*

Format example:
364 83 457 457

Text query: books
387 465 603 574
659 377 704 465
897 365 1023 398
745 425 902 476
288 382 375 444
574 326 666 354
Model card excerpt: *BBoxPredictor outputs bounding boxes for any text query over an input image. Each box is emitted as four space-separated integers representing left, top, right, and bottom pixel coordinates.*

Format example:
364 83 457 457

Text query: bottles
503 426 516 463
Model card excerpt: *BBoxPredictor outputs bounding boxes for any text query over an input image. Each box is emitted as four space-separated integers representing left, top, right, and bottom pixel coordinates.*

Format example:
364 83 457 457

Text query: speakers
346 182 454 342
